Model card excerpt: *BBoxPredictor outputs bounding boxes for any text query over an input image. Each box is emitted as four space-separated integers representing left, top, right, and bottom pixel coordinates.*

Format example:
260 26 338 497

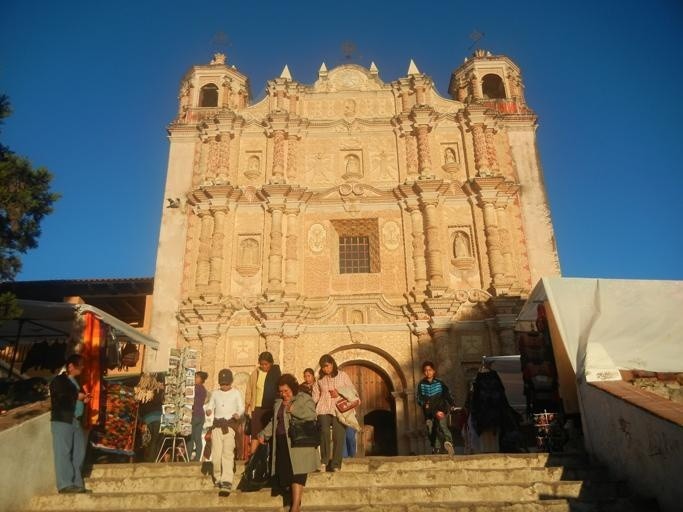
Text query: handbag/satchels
335 393 361 412
288 419 320 447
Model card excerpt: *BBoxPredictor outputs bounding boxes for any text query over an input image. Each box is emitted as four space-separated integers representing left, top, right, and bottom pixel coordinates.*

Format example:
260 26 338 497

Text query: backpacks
244 444 269 485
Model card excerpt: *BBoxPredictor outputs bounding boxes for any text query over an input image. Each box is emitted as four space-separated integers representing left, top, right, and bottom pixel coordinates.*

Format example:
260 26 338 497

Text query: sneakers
319 462 340 474
54 484 93 496
211 480 233 494
432 441 455 457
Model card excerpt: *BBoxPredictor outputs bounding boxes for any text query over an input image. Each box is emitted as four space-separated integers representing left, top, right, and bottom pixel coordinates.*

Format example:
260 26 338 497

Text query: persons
50 354 96 496
416 363 458 458
189 352 362 512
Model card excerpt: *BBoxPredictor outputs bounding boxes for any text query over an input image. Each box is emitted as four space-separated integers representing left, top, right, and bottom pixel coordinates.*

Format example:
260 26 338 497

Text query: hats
218 369 233 385
